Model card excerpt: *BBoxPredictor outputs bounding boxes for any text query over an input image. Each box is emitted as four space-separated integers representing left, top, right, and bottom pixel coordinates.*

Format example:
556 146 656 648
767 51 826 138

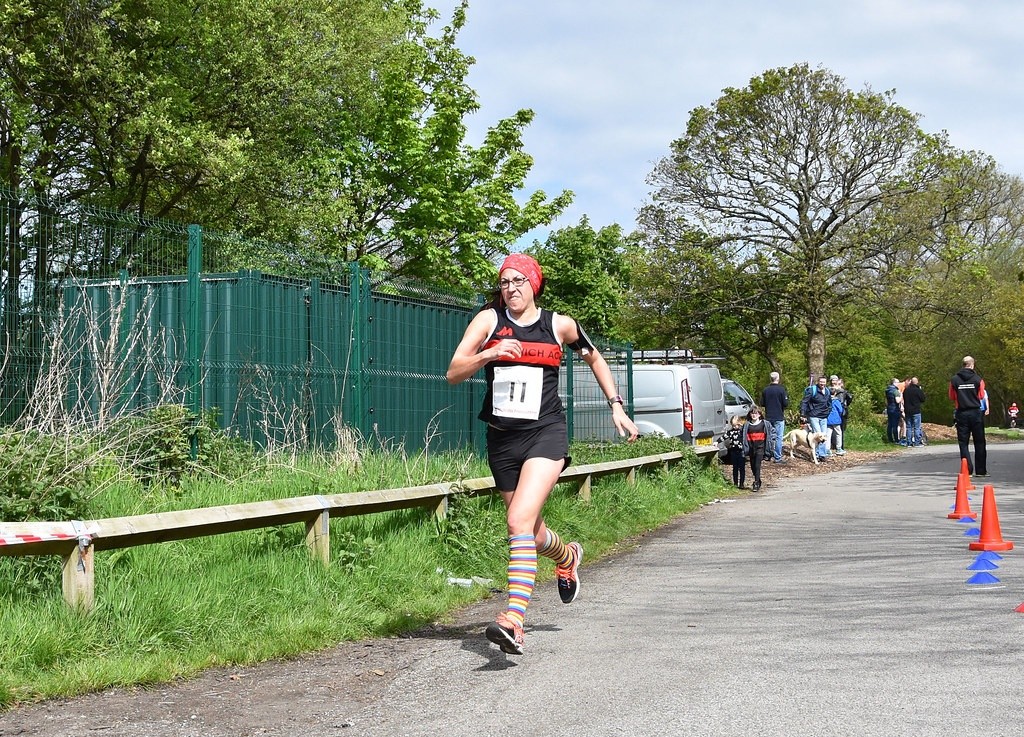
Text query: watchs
607 395 623 408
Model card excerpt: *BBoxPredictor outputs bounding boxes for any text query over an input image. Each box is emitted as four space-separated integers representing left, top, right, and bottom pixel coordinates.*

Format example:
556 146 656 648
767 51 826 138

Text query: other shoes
907 442 914 447
775 457 783 462
739 484 744 489
900 439 907 446
764 454 771 461
894 439 900 444
753 481 761 491
819 456 826 462
914 443 925 447
969 472 974 477
734 482 738 488
976 471 990 476
835 449 846 454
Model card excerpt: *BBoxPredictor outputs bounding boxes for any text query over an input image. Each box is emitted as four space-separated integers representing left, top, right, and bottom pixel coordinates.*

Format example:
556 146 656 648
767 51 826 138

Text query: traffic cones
968 485 1013 551
948 473 978 519
952 458 976 490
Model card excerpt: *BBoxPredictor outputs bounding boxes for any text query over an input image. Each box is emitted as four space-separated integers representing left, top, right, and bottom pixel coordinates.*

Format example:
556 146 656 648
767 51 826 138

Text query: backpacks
804 384 831 398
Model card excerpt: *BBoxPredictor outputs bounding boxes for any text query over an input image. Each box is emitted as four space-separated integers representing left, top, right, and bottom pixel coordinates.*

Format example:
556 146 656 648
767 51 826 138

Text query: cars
721 378 758 464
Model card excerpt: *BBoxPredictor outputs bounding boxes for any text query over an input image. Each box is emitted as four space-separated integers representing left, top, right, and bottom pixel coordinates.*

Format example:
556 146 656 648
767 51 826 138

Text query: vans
555 347 728 473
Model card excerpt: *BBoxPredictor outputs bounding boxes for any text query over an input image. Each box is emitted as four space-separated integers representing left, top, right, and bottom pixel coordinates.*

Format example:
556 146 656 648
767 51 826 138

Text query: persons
1008 403 1019 427
949 356 991 477
952 403 957 427
800 375 848 462
743 406 776 492
885 377 925 447
760 371 789 462
713 415 743 489
979 390 989 416
447 255 637 656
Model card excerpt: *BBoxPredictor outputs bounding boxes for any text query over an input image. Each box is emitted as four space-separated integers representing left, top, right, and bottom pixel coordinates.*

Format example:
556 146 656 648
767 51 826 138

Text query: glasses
497 274 529 289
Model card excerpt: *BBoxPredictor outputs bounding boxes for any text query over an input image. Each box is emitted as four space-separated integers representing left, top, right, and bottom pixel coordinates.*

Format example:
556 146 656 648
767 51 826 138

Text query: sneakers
553 542 584 604
485 611 524 655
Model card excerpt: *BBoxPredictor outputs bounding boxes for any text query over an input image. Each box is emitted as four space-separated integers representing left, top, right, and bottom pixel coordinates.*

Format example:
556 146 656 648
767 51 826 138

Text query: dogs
783 429 827 464
